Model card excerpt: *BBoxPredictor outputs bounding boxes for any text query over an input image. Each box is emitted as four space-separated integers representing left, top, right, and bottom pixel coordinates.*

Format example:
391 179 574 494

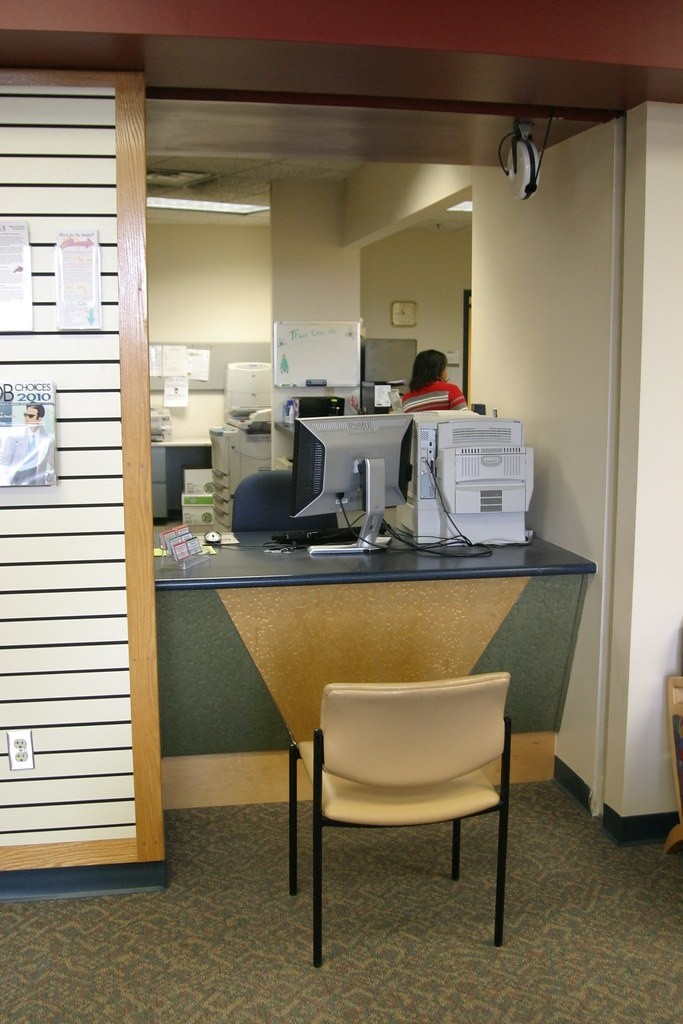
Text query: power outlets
6 728 36 772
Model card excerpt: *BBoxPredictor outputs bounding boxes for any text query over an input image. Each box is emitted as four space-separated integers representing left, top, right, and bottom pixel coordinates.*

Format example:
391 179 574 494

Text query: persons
402 350 469 413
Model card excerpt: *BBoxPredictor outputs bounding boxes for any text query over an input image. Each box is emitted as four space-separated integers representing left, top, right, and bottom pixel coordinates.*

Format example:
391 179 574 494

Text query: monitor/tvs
290 413 415 554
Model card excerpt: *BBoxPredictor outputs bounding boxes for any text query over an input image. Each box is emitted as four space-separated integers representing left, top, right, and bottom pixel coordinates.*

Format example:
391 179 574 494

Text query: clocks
391 299 417 327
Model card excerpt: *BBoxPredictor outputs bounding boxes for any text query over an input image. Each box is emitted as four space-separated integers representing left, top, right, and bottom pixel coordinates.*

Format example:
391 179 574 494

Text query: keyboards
272 524 391 546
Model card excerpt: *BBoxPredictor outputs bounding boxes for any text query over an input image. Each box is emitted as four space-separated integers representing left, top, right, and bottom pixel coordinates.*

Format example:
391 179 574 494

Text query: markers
282 384 296 387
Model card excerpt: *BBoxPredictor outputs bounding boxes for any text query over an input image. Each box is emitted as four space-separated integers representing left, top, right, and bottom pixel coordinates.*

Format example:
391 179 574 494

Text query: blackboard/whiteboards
274 321 359 388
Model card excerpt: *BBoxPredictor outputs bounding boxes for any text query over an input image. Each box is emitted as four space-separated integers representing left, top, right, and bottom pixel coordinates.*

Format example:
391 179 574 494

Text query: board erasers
305 379 327 386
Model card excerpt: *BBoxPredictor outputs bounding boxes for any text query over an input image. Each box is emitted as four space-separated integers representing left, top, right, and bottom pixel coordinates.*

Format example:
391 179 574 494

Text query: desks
155 535 597 590
153 438 211 527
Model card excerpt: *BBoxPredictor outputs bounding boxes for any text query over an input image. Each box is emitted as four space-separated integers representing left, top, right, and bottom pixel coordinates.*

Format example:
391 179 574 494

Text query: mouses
204 531 221 543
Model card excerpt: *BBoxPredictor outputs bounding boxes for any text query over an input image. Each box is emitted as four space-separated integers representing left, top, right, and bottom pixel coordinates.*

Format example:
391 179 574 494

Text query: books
0 382 58 487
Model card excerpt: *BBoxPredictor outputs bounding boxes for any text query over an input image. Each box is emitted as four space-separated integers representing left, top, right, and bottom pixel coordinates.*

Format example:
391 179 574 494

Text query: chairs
233 467 338 532
287 670 514 966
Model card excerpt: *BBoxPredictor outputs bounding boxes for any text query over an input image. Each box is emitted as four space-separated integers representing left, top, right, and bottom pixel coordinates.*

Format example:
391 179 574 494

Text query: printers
208 406 272 532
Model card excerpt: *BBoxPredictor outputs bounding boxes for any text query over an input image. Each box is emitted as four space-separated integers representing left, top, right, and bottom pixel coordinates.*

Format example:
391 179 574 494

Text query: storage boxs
181 491 214 526
182 464 213 496
